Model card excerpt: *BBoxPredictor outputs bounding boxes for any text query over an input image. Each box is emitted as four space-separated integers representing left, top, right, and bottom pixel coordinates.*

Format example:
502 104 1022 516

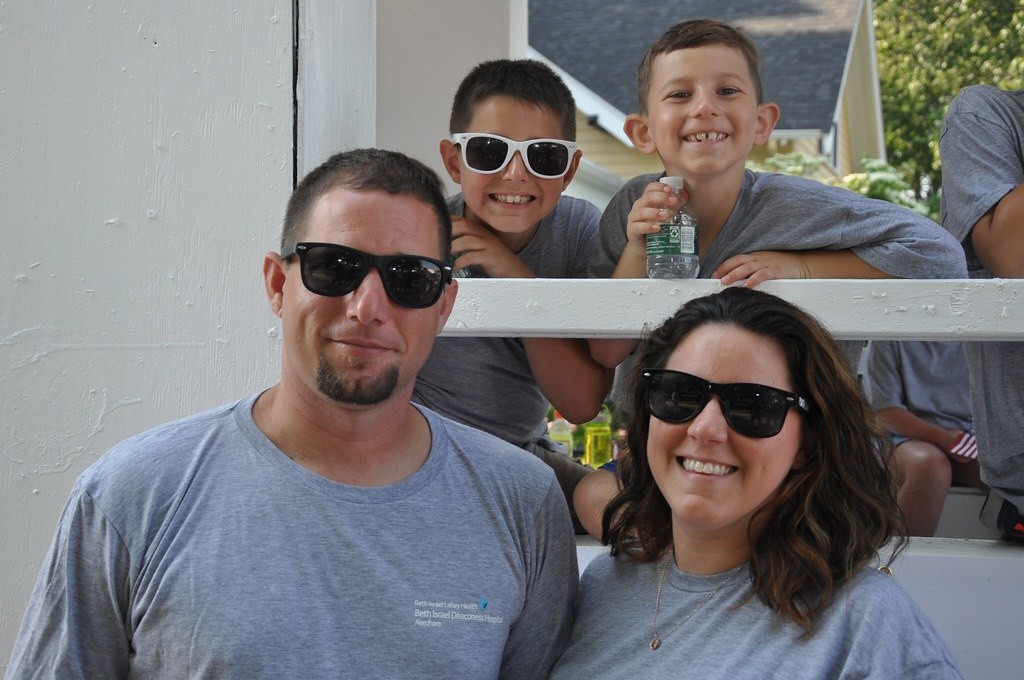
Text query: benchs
438 271 1023 680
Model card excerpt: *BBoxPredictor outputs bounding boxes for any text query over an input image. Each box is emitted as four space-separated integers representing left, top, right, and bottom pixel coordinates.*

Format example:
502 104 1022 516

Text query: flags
948 431 978 461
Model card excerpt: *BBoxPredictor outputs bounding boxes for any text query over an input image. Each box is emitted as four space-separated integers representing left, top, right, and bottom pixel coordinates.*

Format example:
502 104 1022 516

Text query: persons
588 19 968 374
407 60 613 533
548 289 962 679
4 148 579 680
942 85 1024 542
864 341 984 536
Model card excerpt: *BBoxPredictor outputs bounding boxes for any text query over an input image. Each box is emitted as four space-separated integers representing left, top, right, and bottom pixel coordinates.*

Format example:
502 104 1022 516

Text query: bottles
644 175 699 281
545 410 575 459
583 404 613 470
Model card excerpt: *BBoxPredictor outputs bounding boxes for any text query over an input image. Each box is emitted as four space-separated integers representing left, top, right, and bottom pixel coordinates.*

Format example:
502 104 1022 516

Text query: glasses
642 367 811 439
280 242 456 309
451 133 578 180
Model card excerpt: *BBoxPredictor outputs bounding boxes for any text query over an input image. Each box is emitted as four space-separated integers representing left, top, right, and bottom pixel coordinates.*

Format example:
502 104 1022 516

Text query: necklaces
650 545 748 651
